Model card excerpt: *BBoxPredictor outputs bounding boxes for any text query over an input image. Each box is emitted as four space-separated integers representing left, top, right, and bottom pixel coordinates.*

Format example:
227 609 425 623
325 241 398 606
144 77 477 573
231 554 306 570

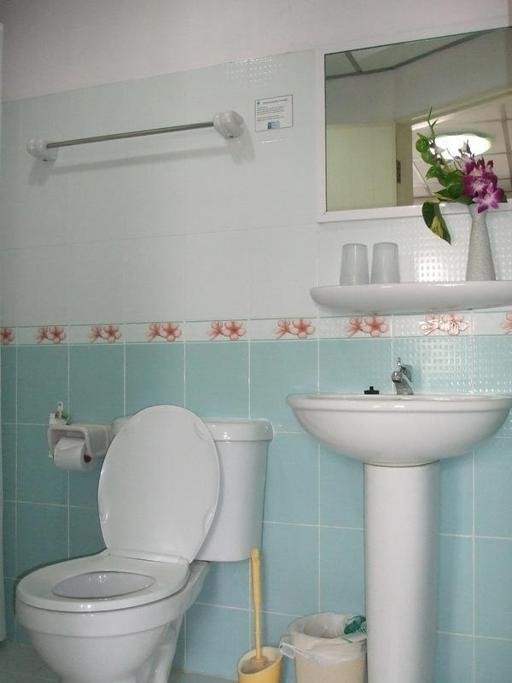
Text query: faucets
391 356 412 395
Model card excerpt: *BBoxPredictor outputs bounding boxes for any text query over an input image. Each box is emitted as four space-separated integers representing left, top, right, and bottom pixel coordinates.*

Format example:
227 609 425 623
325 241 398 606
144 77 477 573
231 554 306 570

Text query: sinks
287 391 512 466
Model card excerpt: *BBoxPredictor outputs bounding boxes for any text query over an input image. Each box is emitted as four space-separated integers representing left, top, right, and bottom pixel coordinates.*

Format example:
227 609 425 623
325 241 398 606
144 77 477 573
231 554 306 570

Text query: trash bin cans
288 612 366 683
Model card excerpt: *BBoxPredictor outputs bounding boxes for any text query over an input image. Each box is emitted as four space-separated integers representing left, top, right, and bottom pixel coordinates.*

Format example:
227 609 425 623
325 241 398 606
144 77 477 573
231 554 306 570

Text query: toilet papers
51 435 92 471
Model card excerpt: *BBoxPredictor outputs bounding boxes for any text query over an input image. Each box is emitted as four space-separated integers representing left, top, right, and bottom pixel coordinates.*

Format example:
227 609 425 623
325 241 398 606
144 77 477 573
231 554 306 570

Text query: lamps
424 131 493 160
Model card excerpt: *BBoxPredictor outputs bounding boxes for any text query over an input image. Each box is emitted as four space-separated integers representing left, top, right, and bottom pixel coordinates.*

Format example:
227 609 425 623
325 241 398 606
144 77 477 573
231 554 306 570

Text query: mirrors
324 26 512 212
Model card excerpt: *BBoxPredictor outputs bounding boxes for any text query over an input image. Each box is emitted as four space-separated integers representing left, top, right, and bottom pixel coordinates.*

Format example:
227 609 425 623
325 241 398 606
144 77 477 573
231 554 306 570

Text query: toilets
12 403 273 683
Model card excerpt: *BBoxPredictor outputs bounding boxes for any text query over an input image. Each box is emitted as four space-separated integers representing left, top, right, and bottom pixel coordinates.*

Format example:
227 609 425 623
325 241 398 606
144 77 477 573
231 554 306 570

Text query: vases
463 200 496 281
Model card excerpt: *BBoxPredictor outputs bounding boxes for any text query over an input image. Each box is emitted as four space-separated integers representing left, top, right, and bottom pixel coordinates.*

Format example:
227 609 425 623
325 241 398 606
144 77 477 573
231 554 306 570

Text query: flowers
415 106 509 244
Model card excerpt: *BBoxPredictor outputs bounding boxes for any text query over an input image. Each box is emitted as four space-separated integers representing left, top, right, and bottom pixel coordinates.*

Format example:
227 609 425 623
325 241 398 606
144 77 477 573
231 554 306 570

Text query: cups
338 242 398 285
